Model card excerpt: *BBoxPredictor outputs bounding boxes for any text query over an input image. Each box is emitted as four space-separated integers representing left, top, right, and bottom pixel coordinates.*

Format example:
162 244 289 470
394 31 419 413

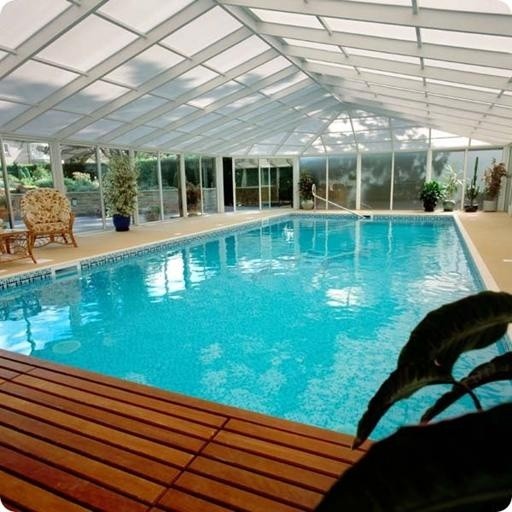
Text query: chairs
20 188 78 250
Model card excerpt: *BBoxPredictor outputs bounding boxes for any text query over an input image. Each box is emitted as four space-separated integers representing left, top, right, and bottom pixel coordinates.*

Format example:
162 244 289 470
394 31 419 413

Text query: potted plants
440 176 458 211
102 152 138 231
298 171 319 209
463 156 480 212
481 162 506 212
419 181 441 211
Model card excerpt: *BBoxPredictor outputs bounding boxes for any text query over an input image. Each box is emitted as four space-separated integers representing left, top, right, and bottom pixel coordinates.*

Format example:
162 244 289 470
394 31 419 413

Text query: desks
0 228 37 264
